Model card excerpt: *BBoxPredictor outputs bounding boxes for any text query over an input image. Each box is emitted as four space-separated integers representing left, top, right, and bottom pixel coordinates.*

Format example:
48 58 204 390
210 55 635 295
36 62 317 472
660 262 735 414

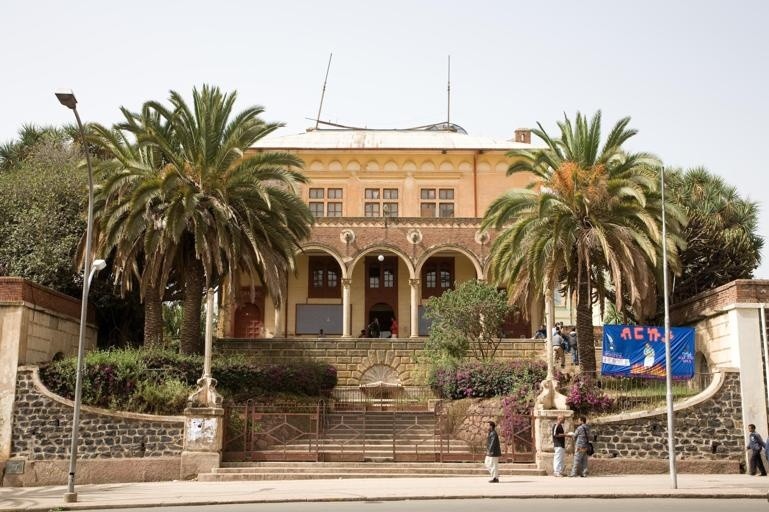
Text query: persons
747 424 766 476
644 343 655 369
485 421 502 482
552 415 591 477
391 316 398 338
359 318 380 338
531 322 577 367
318 330 326 338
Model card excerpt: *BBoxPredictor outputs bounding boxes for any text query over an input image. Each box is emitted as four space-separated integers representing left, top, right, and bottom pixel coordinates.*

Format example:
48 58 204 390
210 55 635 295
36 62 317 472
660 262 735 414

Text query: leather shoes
488 478 498 482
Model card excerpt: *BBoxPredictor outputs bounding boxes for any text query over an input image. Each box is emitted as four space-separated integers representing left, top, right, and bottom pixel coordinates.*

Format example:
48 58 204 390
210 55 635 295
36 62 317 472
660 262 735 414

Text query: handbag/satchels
588 443 593 456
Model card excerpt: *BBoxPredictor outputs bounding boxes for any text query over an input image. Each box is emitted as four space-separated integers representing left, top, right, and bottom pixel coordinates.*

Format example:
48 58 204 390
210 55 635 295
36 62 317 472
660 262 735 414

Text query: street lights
55 88 106 503
